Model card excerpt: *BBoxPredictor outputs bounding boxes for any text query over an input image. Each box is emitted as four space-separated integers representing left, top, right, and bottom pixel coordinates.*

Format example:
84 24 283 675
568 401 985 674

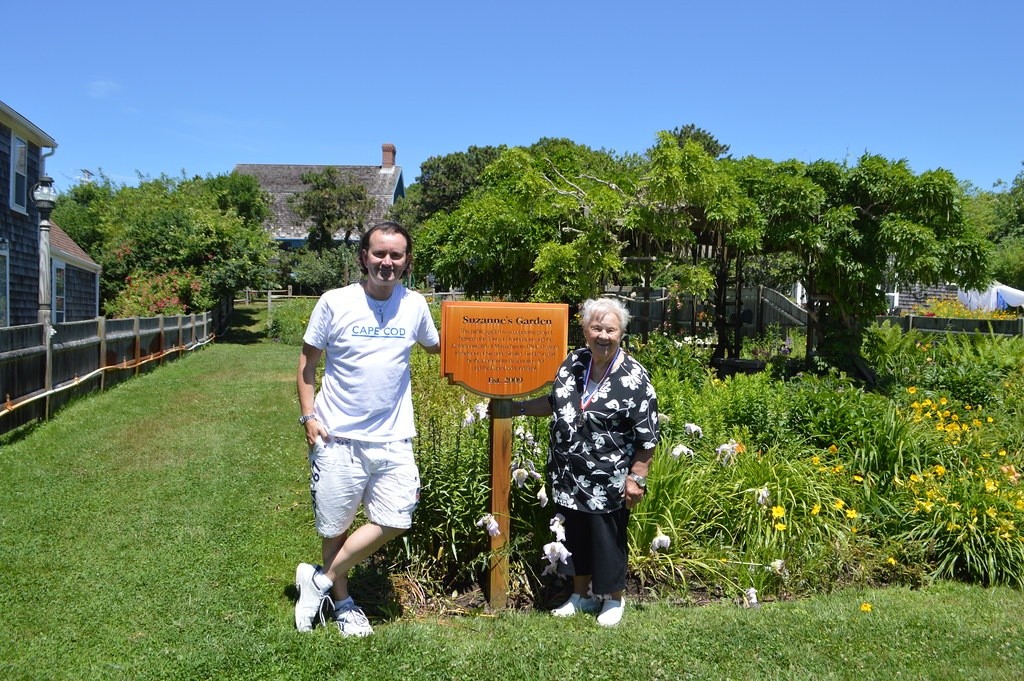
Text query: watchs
630 472 648 493
298 414 317 424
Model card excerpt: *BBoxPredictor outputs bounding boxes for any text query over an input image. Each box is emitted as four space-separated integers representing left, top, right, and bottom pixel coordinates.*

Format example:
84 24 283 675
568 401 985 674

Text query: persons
485 297 659 625
295 223 441 639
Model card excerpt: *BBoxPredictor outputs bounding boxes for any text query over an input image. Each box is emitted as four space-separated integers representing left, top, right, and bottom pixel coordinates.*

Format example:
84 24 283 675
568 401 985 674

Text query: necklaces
365 290 393 322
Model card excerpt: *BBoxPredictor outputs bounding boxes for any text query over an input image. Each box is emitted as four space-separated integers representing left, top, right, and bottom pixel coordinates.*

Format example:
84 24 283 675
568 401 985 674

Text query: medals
574 411 587 427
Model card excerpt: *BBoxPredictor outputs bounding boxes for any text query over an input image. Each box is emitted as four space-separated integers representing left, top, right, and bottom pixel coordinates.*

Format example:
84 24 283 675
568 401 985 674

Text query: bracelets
520 401 526 415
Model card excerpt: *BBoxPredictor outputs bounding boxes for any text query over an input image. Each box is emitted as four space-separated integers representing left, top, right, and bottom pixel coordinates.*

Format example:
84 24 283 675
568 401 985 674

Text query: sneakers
295 563 335 633
550 593 601 617
597 596 625 627
326 596 375 639
